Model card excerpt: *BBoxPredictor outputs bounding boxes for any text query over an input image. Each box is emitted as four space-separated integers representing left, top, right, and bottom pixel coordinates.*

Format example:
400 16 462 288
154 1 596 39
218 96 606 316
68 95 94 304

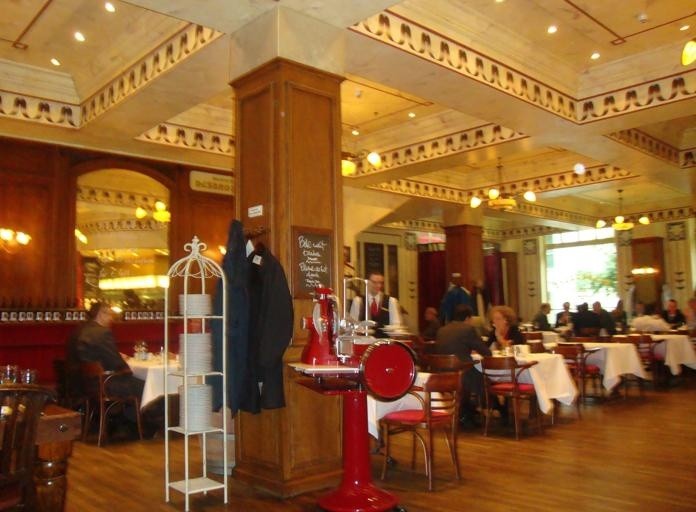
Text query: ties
371 298 378 317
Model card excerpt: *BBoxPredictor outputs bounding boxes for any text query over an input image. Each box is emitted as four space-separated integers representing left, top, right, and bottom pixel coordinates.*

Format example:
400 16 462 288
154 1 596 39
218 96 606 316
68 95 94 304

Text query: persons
434 303 492 430
469 273 493 316
531 297 696 337
486 306 525 423
422 305 441 337
348 270 402 338
64 300 158 441
439 272 473 325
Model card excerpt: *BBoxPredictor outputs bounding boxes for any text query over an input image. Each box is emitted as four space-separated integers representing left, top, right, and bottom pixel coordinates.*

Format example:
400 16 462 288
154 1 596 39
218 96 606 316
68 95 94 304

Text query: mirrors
70 158 179 316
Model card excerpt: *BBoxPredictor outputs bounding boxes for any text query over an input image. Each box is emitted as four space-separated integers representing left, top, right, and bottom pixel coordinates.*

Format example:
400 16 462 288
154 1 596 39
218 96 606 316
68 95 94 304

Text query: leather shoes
459 415 473 429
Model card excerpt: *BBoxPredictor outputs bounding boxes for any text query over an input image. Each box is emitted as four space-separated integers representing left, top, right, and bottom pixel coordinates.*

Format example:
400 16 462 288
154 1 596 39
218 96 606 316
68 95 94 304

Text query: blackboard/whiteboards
291 225 334 299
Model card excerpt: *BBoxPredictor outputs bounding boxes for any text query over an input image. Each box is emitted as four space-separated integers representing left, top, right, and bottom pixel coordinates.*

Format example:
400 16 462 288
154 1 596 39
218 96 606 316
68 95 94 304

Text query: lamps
341 147 382 177
680 36 696 66
0 225 32 255
470 157 536 212
596 189 650 231
133 189 172 223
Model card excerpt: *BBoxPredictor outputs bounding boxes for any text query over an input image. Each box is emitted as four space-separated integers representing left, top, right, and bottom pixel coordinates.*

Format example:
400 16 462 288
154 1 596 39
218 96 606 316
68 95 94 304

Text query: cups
514 344 532 359
0 365 36 384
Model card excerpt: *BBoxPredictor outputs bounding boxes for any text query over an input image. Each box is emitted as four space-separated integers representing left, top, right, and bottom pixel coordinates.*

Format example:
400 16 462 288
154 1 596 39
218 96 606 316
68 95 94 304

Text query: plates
178 332 214 375
380 325 412 338
178 385 212 431
179 292 214 316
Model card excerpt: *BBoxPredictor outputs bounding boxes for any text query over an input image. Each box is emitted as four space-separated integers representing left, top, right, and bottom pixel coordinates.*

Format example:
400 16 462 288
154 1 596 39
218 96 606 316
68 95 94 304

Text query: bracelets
495 336 504 340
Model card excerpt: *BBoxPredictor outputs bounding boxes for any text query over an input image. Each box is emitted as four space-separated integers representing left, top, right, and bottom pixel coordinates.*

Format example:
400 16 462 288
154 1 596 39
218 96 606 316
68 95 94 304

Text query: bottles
0 295 85 322
122 295 164 319
500 343 514 355
134 346 165 363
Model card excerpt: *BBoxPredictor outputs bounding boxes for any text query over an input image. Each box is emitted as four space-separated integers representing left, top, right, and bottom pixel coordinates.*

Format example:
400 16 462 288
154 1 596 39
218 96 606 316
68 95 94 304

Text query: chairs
0 382 54 512
78 358 145 448
55 362 95 435
